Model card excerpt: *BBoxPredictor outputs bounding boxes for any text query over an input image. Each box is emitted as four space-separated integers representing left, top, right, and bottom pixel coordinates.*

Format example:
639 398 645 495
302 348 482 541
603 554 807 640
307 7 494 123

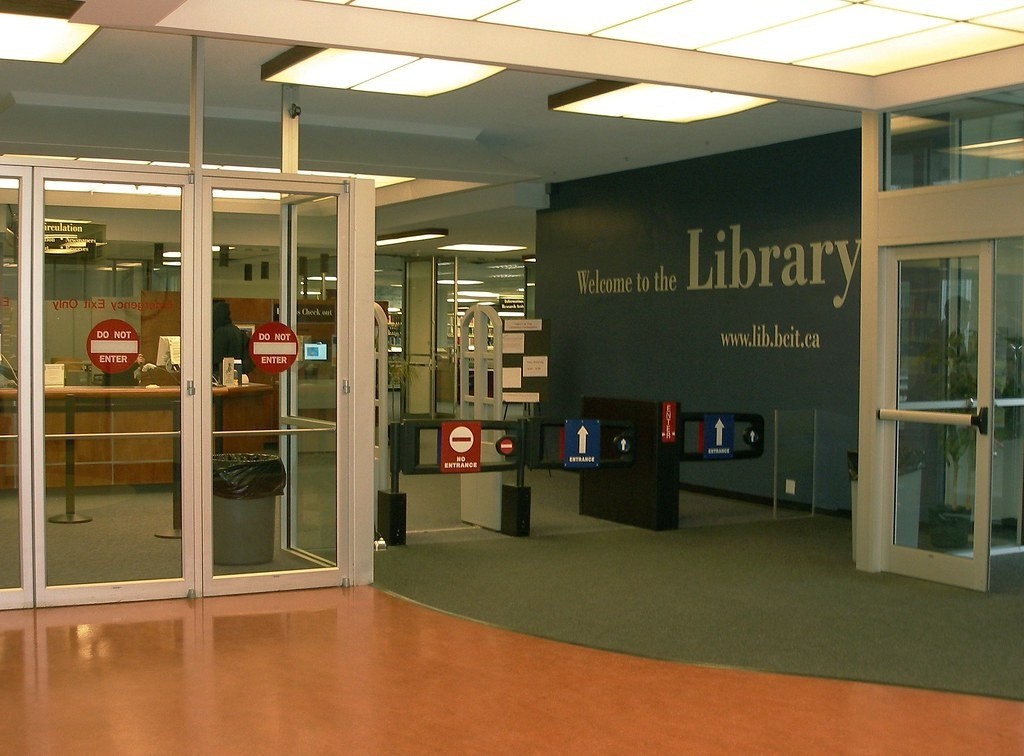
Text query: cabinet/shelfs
447 314 494 338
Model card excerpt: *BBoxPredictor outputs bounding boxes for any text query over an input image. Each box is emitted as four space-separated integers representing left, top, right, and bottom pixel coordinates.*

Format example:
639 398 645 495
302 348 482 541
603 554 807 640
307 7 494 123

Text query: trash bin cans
210 452 280 565
847 445 925 564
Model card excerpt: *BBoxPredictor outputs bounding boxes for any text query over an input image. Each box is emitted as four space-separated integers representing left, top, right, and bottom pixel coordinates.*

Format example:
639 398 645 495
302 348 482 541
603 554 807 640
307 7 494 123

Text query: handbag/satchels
230 328 257 375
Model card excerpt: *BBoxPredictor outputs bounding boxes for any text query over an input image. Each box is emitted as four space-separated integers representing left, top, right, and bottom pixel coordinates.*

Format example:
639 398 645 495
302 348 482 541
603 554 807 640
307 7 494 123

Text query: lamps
375 228 448 247
0 0 102 65
547 73 780 124
261 46 507 97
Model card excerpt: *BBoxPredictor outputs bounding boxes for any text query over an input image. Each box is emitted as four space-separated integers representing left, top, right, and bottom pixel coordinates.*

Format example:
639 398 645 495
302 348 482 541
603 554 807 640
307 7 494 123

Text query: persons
211 299 257 384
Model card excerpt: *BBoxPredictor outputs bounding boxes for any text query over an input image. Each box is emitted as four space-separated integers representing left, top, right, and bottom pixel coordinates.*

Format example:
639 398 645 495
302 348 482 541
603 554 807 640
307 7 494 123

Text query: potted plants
911 326 1018 547
